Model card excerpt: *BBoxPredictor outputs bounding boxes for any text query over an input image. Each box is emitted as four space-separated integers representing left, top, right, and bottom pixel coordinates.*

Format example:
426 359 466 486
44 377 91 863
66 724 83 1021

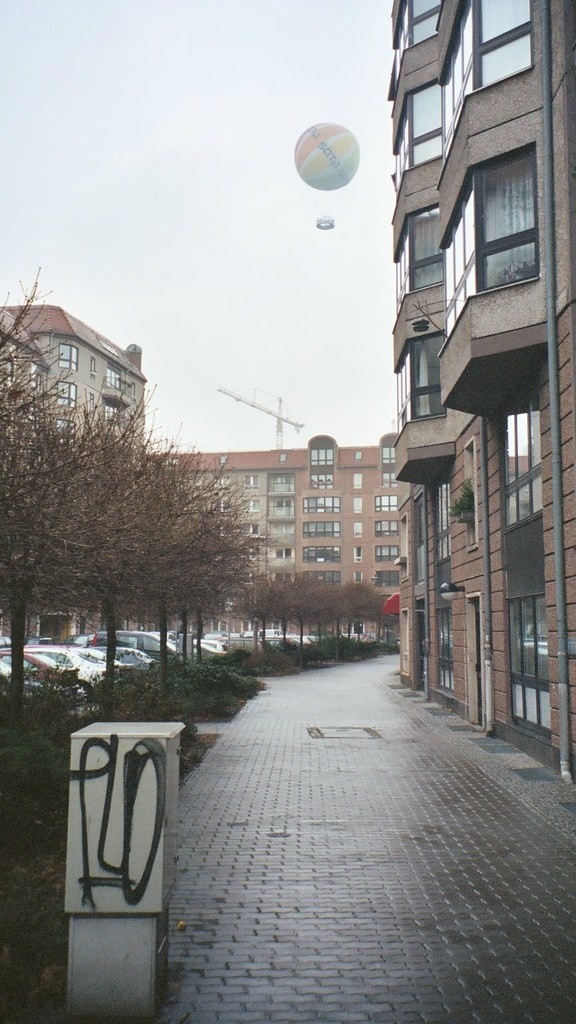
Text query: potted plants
448 482 475 523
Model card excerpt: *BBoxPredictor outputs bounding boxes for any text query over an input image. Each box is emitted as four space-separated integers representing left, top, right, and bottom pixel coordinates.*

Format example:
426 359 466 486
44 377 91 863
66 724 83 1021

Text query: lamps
438 582 465 602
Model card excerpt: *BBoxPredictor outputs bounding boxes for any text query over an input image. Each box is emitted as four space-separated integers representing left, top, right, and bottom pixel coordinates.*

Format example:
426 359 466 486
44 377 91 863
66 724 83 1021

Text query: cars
1 628 379 707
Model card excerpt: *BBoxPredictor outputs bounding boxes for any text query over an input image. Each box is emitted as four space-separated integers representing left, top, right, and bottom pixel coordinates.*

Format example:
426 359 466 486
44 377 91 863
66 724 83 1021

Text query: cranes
216 386 306 449
